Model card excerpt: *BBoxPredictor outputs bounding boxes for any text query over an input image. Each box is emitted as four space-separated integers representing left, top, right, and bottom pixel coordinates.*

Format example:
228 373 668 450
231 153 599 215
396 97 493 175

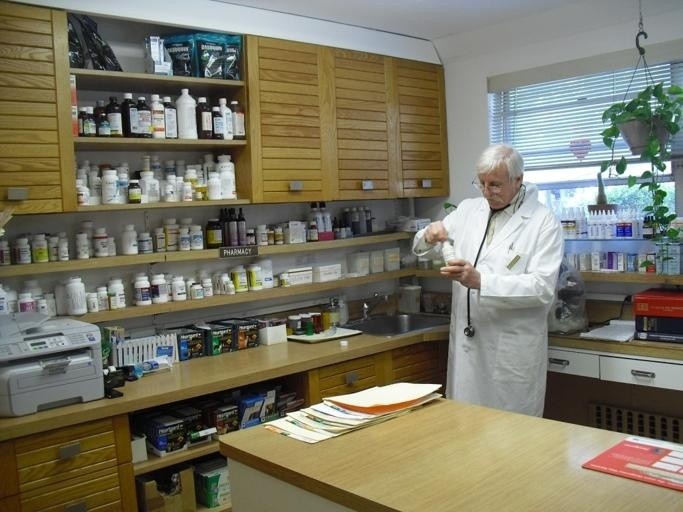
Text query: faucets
361 294 387 319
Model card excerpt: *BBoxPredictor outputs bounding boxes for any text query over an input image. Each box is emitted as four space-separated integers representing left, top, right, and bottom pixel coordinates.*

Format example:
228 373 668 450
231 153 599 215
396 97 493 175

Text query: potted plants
600 81 683 278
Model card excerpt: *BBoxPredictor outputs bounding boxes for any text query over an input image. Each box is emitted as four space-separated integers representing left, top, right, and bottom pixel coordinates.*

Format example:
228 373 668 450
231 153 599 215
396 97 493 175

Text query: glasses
470 174 510 195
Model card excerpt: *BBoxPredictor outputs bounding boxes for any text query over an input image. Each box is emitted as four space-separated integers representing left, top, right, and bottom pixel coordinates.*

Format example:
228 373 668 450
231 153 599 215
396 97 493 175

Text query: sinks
343 310 451 338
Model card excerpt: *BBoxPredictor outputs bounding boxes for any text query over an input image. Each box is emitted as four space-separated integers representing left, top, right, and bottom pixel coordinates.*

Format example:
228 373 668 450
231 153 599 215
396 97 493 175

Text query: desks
219 398 683 512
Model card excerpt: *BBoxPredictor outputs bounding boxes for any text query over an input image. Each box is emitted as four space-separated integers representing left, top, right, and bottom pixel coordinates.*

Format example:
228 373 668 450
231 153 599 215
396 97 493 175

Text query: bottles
75 149 238 207
219 98 233 140
195 96 213 139
76 90 165 140
175 88 198 140
162 94 177 139
442 240 457 267
211 106 225 140
229 101 246 140
551 206 664 240
285 294 349 336
0 202 382 316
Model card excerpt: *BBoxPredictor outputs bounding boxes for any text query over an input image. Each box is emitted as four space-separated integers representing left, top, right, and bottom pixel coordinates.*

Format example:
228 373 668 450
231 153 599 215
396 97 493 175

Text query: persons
409 143 566 421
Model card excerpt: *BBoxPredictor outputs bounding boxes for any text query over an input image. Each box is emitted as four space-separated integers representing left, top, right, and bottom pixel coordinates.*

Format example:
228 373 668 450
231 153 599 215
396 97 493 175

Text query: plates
286 327 362 344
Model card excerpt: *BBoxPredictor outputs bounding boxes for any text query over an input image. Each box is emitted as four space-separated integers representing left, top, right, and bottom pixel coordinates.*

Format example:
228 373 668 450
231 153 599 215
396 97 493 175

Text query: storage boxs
136 456 231 512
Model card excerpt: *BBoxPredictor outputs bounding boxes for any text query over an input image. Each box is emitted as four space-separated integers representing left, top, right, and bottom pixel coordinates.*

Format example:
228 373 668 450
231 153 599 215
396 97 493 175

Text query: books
579 318 636 343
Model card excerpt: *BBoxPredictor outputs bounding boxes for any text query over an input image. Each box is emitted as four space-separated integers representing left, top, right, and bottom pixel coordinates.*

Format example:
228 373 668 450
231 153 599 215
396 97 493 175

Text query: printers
1 314 106 417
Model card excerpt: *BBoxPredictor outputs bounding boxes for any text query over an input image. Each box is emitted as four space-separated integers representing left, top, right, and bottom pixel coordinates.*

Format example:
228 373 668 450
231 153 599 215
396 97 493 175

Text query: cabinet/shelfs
307 340 449 409
247 35 332 205
0 229 418 323
565 238 683 285
544 347 683 433
1 0 70 216
1 413 138 512
325 46 449 200
129 362 309 512
66 10 249 213
